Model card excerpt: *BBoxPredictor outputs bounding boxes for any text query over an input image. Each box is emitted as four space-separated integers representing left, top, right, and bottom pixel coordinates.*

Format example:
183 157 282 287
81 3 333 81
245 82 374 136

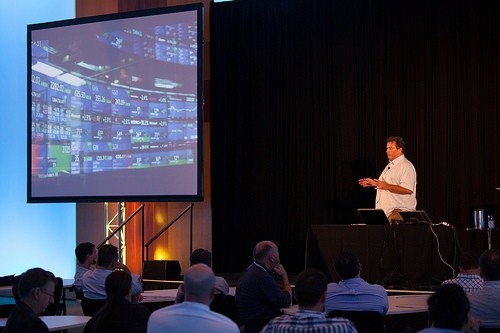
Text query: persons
175 248 229 304
465 249 500 328
72 242 97 302
259 268 359 333
90 270 151 333
2 268 57 333
358 136 417 223
147 263 240 333
440 252 484 296
322 252 388 318
416 283 480 333
82 244 144 303
236 241 292 333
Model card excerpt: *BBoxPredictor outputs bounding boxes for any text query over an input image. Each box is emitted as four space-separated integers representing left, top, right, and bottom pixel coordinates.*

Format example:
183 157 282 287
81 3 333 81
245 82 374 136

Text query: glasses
113 268 126 272
41 289 54 299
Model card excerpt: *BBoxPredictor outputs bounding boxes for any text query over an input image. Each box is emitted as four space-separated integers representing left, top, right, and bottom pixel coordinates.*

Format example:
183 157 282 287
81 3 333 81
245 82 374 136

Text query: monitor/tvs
398 210 432 224
358 209 391 226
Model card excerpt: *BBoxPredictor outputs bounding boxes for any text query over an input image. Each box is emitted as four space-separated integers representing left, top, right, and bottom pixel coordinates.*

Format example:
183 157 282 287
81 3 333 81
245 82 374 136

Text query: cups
474 209 484 230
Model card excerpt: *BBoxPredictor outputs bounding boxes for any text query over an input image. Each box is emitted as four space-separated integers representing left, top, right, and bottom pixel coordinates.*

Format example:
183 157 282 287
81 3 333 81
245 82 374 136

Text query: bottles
488 214 495 229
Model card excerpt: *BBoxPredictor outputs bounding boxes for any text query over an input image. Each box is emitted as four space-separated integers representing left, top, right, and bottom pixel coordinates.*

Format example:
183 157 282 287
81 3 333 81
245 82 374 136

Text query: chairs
39 277 105 317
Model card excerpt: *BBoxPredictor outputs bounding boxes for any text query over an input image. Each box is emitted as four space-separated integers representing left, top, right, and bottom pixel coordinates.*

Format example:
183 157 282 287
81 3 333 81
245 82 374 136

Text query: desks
280 295 430 316
138 286 236 303
305 224 500 291
0 316 92 331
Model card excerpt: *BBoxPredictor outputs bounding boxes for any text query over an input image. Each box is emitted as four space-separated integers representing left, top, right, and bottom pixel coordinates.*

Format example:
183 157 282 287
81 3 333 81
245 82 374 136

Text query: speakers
142 260 182 291
